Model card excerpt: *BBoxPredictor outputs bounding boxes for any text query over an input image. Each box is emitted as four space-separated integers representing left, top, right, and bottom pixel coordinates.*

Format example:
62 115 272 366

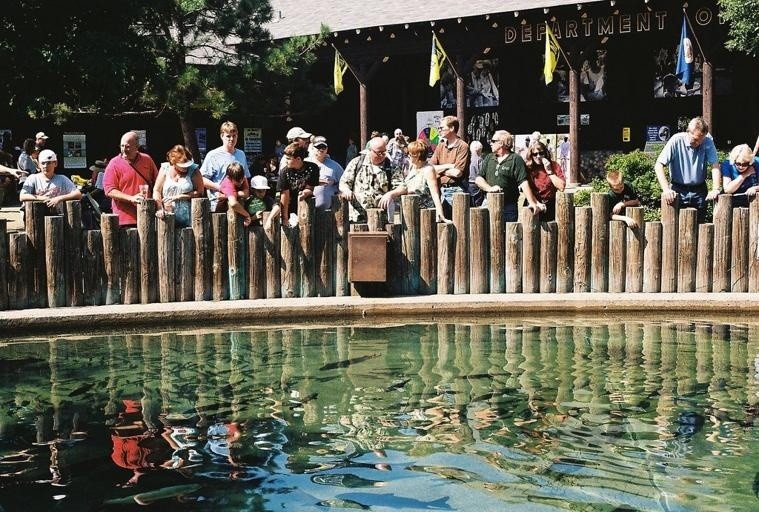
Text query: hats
35 131 49 141
174 148 195 168
312 135 328 147
37 148 59 163
287 127 313 141
89 159 107 173
251 174 271 191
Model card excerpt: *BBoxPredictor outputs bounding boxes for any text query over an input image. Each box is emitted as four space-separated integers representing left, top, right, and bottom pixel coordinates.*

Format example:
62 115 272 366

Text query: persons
0 119 344 230
655 117 722 227
721 143 758 208
338 115 566 228
607 171 640 229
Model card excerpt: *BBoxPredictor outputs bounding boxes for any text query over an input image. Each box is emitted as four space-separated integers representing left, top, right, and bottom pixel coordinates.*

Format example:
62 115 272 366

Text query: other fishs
318 374 343 383
102 482 203 503
0 342 319 423
319 352 377 371
316 490 455 512
386 371 759 484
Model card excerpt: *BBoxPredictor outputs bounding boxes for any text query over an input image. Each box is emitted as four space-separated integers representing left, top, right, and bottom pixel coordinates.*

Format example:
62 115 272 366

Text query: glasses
314 144 327 150
531 151 546 156
735 161 751 168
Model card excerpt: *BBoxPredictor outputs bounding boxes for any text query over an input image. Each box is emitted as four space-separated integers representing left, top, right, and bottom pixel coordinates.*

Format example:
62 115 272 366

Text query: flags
673 15 700 92
329 49 350 97
540 26 564 85
427 34 448 89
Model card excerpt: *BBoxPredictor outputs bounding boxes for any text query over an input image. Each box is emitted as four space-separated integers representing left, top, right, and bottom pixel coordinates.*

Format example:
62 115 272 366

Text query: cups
139 185 149 199
